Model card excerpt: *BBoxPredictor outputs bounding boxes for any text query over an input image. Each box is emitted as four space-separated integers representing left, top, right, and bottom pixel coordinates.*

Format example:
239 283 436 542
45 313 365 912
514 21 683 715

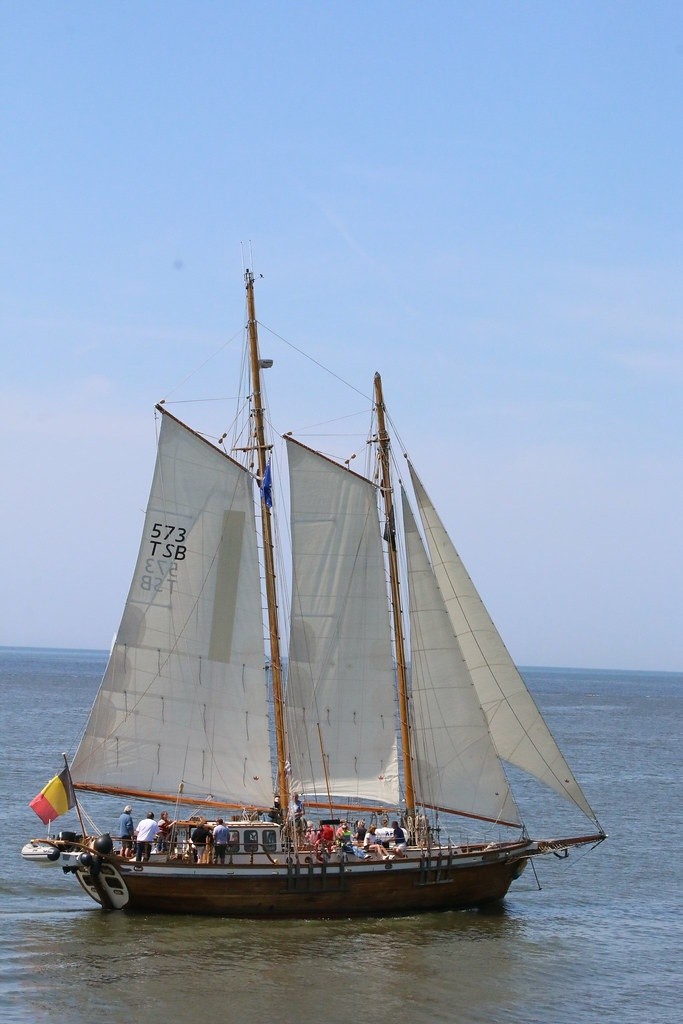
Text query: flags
28 765 77 825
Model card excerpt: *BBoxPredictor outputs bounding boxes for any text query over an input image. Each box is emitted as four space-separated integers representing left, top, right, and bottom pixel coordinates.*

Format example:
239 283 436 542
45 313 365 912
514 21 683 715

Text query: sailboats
21 241 609 918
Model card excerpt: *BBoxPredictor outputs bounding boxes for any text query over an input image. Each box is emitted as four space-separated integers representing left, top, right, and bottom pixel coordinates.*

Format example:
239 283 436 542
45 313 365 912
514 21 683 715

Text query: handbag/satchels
345 842 353 853
382 841 389 848
374 839 382 845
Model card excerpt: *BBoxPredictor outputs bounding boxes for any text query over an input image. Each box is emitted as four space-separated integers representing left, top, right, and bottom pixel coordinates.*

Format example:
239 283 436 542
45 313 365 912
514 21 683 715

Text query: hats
124 806 132 813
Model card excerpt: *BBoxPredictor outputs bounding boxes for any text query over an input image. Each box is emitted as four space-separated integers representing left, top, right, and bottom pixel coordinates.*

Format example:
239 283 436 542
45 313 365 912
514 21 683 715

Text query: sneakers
389 854 395 860
383 855 389 861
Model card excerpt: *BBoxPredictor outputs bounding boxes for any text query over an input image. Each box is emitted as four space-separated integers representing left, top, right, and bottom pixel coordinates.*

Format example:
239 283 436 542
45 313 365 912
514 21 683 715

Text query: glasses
342 828 346 830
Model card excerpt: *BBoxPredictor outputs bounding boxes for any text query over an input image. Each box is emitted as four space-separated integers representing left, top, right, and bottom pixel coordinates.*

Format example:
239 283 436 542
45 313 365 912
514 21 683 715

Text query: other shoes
364 855 373 859
403 854 408 858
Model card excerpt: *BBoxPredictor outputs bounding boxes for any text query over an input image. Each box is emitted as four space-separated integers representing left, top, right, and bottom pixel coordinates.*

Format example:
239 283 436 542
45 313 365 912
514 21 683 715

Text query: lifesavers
314 838 332 862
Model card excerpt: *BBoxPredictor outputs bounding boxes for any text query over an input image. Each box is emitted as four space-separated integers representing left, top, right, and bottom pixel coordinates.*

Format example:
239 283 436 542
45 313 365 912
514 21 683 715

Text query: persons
136 812 160 862
157 791 408 864
117 805 134 857
